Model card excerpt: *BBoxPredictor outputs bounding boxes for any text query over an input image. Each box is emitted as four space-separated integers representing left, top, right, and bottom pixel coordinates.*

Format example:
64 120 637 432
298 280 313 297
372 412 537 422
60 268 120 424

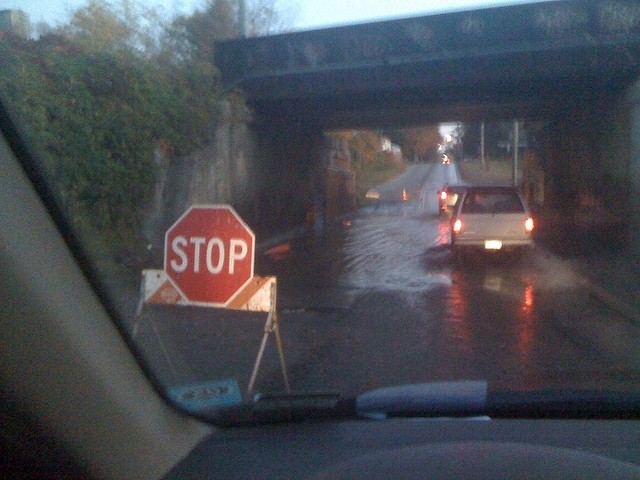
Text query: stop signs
162 202 256 308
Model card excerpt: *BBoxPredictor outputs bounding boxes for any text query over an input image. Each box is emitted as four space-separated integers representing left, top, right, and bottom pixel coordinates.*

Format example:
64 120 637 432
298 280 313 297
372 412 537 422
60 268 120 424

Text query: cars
437 183 470 217
446 184 536 255
